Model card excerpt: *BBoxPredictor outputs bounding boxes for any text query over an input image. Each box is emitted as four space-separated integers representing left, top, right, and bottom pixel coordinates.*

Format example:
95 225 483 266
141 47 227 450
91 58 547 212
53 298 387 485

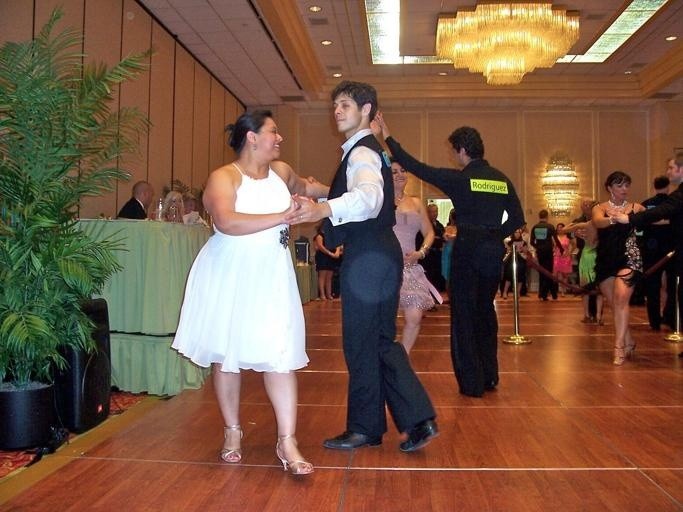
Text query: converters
47 427 68 450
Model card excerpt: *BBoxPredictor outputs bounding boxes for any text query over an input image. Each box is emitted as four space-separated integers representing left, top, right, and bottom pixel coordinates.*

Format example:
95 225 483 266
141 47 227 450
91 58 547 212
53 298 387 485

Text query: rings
301 215 303 220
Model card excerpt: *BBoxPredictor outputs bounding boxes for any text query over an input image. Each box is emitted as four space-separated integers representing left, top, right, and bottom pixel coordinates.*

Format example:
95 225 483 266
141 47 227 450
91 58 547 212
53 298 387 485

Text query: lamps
434 0 579 85
541 150 581 218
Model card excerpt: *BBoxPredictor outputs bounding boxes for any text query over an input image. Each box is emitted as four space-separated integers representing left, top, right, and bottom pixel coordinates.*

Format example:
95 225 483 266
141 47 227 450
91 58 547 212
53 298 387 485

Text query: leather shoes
322 430 382 448
400 420 438 451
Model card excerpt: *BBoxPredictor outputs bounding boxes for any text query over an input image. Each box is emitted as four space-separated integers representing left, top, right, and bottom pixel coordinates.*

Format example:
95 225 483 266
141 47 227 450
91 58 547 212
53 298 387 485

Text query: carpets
1 389 147 485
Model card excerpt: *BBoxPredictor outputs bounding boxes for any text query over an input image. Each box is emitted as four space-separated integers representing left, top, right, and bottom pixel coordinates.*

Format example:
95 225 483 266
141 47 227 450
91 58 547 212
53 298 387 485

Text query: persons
116 181 199 219
376 111 524 397
500 153 683 366
312 224 344 301
415 204 458 312
370 110 443 355
171 109 329 475
285 81 440 451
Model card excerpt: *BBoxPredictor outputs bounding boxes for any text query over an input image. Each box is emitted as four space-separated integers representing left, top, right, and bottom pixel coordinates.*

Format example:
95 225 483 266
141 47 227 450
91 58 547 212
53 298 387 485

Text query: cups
155 195 177 224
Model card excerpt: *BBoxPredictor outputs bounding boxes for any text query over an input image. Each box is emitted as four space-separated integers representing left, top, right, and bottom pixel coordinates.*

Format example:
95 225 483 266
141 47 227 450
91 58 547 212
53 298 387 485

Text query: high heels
612 340 637 366
276 434 314 474
580 316 604 325
221 424 243 463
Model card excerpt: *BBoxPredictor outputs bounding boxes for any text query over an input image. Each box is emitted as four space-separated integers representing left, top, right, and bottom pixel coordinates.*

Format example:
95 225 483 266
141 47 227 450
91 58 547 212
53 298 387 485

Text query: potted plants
0 6 160 449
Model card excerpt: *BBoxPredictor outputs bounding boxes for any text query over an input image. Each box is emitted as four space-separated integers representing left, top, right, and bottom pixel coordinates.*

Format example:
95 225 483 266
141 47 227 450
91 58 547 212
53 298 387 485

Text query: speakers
8 298 111 434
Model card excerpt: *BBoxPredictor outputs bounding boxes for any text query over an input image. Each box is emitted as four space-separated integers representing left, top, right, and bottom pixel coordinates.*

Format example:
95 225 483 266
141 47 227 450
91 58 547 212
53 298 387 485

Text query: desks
297 265 319 305
61 218 213 396
